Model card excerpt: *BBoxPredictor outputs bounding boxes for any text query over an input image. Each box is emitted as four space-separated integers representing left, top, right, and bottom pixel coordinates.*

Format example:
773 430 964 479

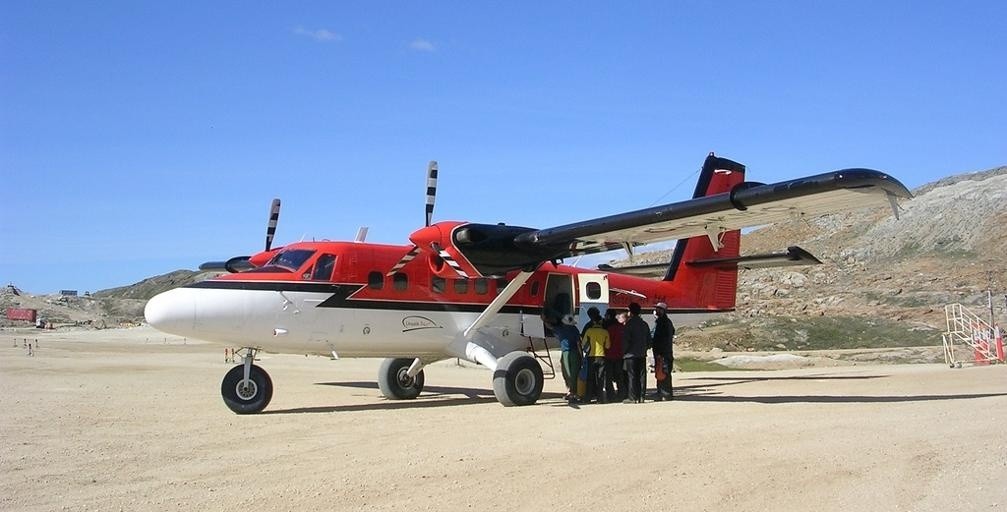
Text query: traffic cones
966 320 1006 369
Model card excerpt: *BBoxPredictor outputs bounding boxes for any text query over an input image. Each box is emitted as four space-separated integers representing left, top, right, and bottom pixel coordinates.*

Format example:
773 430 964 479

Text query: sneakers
562 395 672 403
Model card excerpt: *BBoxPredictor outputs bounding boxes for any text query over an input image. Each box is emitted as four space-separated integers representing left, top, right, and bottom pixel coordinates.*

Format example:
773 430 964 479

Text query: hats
651 303 667 309
562 314 577 326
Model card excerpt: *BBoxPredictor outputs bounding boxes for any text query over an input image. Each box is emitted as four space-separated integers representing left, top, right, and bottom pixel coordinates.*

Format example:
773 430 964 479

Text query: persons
602 308 628 399
617 312 628 325
577 305 602 332
647 301 676 400
575 314 613 400
540 309 583 400
617 301 652 404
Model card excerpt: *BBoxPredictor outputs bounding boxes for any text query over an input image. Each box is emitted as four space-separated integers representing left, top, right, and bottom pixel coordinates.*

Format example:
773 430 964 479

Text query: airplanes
139 152 912 417
